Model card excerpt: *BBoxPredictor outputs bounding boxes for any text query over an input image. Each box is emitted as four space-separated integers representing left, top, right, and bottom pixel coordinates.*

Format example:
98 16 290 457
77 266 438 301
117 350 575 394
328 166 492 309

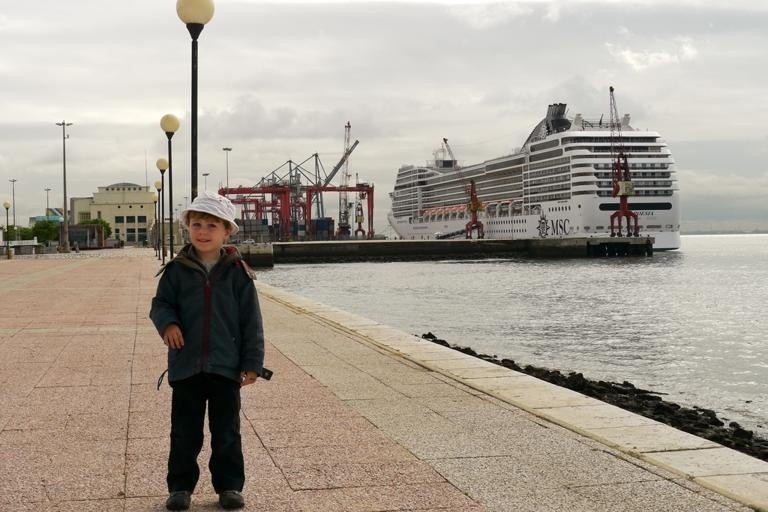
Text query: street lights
202 171 210 193
150 194 158 257
149 218 159 250
221 146 232 201
13 225 18 241
9 178 18 241
153 179 162 261
156 157 168 264
44 188 53 248
173 193 189 244
58 216 65 248
176 1 218 246
56 118 74 251
161 114 180 259
3 202 12 258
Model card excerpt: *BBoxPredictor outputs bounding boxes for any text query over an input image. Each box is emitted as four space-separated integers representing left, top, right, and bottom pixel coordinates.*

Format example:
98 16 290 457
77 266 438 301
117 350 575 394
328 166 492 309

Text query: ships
386 100 685 257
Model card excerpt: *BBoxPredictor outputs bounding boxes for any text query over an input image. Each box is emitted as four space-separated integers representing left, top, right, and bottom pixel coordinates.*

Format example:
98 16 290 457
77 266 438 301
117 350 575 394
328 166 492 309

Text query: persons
147 188 274 510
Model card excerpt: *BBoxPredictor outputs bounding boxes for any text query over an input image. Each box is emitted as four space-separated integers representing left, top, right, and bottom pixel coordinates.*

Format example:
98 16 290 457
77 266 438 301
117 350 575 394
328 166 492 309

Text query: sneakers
220 491 244 509
166 491 191 511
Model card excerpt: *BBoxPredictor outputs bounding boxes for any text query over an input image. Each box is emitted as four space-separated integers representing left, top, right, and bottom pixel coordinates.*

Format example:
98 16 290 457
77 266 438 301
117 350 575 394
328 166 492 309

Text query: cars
227 238 256 245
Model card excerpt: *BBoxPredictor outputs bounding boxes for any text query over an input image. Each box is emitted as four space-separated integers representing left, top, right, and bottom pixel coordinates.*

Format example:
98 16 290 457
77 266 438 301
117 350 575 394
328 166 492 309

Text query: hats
180 192 240 236
